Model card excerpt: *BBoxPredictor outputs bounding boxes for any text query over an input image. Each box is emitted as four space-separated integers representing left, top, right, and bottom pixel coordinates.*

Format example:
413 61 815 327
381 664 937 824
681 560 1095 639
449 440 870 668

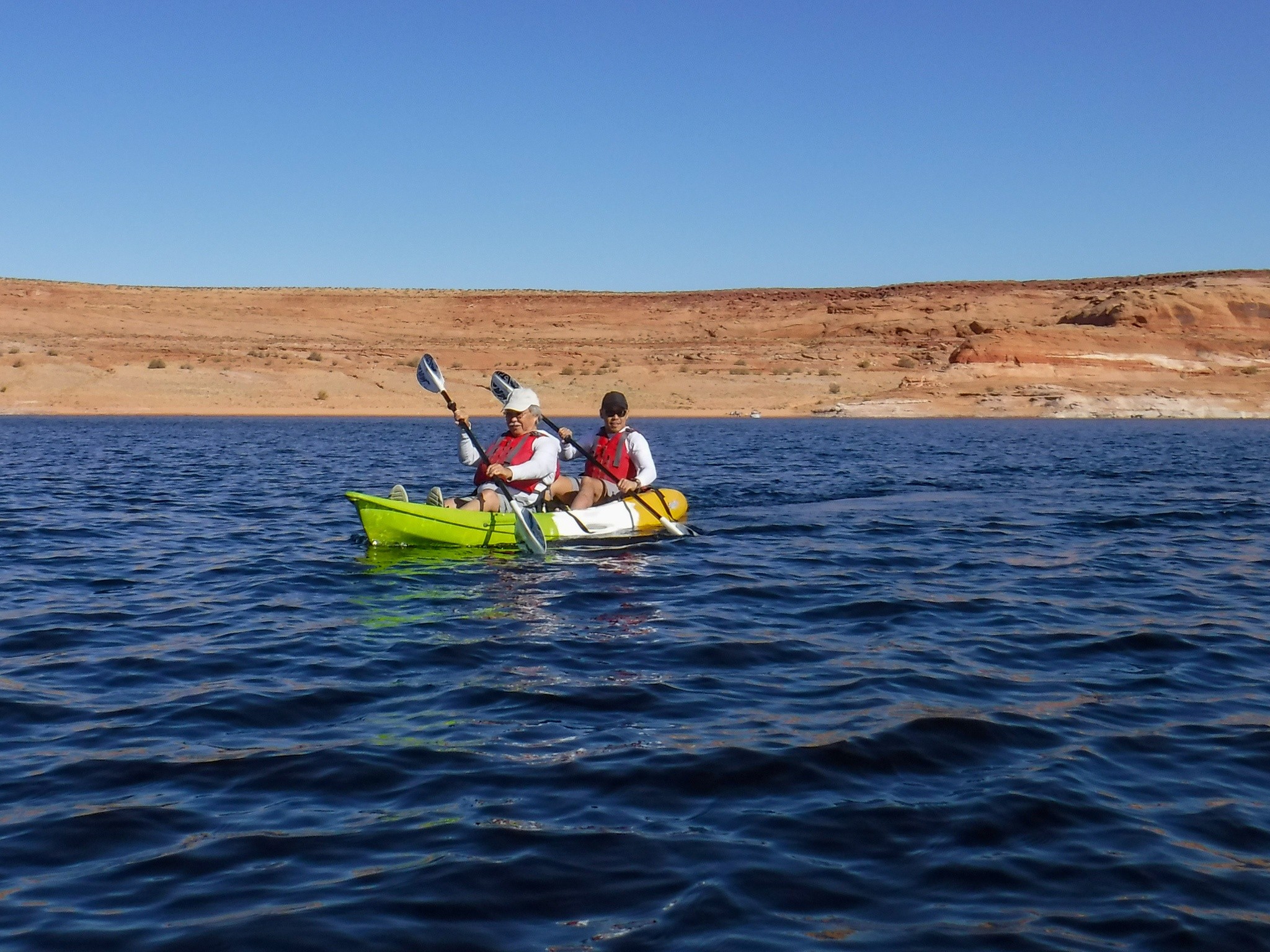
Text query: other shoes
426 487 444 507
554 504 572 512
389 484 409 502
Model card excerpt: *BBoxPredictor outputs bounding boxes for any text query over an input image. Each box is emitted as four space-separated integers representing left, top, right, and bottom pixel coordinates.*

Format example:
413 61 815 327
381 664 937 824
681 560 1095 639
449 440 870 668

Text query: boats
343 488 690 547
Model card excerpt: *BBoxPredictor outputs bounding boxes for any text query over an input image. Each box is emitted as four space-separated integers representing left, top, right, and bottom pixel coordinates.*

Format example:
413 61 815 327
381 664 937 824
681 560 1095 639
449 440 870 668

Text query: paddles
490 370 699 536
417 353 547 555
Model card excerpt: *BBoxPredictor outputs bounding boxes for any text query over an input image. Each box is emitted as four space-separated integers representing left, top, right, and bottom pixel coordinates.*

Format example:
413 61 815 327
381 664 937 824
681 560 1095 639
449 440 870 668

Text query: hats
602 391 628 409
501 388 540 412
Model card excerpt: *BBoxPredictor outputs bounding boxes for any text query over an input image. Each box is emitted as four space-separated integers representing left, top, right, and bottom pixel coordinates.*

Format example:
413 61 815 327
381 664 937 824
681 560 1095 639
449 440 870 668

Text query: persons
543 391 657 509
388 388 561 513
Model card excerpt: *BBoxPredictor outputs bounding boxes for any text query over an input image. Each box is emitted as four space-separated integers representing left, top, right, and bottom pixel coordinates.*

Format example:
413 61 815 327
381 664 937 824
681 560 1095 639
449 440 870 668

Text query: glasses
503 411 529 419
604 408 626 417
